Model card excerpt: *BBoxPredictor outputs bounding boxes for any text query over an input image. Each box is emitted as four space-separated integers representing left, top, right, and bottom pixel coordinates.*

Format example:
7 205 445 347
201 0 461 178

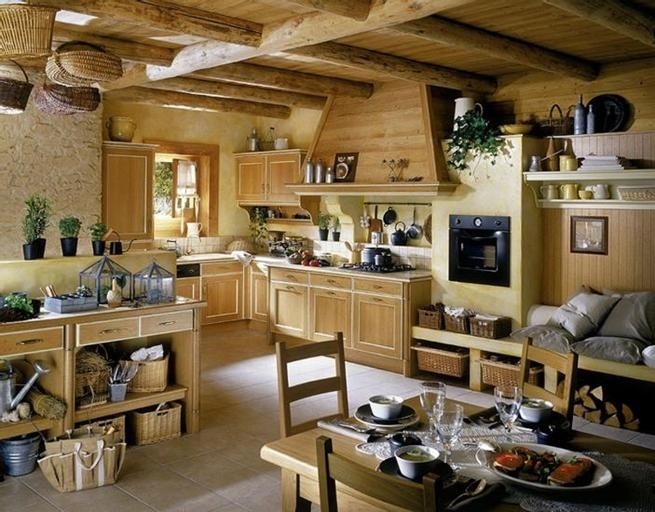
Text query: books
577 152 637 170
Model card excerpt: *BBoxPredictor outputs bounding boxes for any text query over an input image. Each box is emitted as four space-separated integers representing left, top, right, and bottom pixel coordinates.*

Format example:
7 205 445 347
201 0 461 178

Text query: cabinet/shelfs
352 274 432 378
249 260 271 336
308 265 352 364
235 148 320 225
174 257 246 325
270 262 308 340
1 317 70 450
101 139 155 238
69 300 203 445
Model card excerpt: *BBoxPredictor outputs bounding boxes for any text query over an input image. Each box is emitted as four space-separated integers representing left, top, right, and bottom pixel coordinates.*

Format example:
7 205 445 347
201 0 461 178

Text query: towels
446 483 506 510
317 418 389 444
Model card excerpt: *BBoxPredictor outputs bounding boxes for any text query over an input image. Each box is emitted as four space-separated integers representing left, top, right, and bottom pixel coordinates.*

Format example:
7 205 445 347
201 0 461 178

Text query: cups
539 182 580 200
185 221 203 237
559 155 578 171
273 138 289 149
590 182 610 200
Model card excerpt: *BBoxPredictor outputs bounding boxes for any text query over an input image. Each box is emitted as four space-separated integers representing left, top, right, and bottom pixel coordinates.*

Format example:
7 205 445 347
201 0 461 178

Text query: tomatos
302 249 312 257
301 259 320 266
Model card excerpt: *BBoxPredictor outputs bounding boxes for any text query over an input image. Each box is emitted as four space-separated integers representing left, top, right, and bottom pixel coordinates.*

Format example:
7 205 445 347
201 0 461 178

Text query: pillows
510 320 576 355
541 283 615 335
598 288 655 343
568 333 642 365
641 342 655 368
526 302 561 330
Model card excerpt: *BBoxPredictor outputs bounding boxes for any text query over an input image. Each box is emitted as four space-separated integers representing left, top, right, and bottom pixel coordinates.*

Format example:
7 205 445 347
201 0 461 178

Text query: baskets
443 310 477 334
479 355 543 395
469 314 512 338
134 402 182 445
119 352 169 393
410 342 470 378
76 345 110 405
535 105 575 136
0 3 124 116
418 305 451 330
618 188 655 202
78 415 126 442
225 238 259 254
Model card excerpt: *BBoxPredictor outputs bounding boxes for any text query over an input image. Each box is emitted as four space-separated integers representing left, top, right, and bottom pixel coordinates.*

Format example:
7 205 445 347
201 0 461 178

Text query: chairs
275 329 350 442
513 335 580 430
313 434 443 511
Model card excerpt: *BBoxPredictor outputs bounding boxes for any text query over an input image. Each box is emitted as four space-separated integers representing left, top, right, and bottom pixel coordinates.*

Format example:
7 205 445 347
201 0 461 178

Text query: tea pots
345 241 362 264
389 223 408 246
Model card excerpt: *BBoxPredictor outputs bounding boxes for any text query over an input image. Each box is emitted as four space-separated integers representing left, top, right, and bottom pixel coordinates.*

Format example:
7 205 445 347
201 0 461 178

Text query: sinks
176 253 235 263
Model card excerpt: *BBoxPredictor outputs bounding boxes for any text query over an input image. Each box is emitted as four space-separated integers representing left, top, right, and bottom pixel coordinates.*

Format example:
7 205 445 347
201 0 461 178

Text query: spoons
445 475 505 510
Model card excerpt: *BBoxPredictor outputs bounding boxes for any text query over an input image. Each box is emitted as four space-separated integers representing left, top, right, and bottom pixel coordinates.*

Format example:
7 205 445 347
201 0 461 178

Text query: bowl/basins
576 190 594 201
516 397 554 424
369 394 404 419
393 444 442 478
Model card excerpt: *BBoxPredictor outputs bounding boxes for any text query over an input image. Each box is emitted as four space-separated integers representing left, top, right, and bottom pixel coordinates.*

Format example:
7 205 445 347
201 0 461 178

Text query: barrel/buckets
245 137 262 152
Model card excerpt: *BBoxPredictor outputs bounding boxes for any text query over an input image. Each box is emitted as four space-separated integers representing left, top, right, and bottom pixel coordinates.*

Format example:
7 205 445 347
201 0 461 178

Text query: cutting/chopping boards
366 206 381 243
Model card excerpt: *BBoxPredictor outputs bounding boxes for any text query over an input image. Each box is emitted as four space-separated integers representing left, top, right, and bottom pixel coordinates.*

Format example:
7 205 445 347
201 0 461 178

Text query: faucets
185 235 202 253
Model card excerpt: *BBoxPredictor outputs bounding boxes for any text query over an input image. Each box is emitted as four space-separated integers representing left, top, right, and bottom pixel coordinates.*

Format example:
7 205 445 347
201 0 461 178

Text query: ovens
448 214 510 288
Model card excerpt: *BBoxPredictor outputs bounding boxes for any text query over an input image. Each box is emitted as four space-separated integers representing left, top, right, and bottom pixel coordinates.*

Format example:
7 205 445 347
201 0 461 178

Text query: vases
105 115 139 146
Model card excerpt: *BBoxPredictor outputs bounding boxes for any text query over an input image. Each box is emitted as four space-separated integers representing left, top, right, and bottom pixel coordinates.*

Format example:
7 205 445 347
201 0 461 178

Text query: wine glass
492 383 522 443
417 380 464 467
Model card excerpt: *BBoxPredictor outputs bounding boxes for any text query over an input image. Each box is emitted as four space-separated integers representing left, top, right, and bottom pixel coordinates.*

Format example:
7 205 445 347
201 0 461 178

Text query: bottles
247 127 257 151
587 103 596 134
529 137 558 172
303 156 333 184
573 93 585 136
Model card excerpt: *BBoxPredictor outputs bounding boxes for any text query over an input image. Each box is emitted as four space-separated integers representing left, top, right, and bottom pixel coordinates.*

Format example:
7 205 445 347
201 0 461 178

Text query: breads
548 457 594 487
493 453 521 474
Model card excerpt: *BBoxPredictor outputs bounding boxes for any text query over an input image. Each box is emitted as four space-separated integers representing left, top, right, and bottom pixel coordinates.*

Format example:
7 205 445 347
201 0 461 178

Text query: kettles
453 98 484 137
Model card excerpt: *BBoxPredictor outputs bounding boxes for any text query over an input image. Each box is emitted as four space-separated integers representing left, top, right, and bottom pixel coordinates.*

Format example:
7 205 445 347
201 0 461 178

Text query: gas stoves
339 263 416 275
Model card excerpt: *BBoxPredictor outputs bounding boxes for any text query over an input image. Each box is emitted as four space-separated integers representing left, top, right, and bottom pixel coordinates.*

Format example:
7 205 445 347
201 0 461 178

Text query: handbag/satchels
37 425 127 494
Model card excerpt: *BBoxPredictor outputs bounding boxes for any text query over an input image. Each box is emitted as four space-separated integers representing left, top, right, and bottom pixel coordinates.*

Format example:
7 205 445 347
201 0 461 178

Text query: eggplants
320 260 330 267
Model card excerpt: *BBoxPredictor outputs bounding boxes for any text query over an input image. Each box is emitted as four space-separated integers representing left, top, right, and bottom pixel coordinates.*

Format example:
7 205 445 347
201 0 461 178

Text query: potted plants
88 220 108 256
21 191 48 262
330 213 342 243
57 213 82 257
317 209 329 241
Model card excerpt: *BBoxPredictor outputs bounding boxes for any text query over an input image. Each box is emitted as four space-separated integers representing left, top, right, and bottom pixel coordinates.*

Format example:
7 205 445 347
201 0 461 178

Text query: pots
408 208 423 241
359 247 393 266
381 206 398 227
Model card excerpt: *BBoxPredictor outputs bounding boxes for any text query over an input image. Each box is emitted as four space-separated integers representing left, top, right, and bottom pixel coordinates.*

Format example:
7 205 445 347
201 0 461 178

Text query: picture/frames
331 151 360 182
569 214 611 255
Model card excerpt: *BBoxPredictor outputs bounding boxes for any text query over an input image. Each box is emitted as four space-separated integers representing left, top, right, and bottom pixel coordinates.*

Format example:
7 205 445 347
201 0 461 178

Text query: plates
586 94 633 133
353 404 420 431
378 456 458 487
338 163 347 179
497 124 532 135
473 441 612 491
